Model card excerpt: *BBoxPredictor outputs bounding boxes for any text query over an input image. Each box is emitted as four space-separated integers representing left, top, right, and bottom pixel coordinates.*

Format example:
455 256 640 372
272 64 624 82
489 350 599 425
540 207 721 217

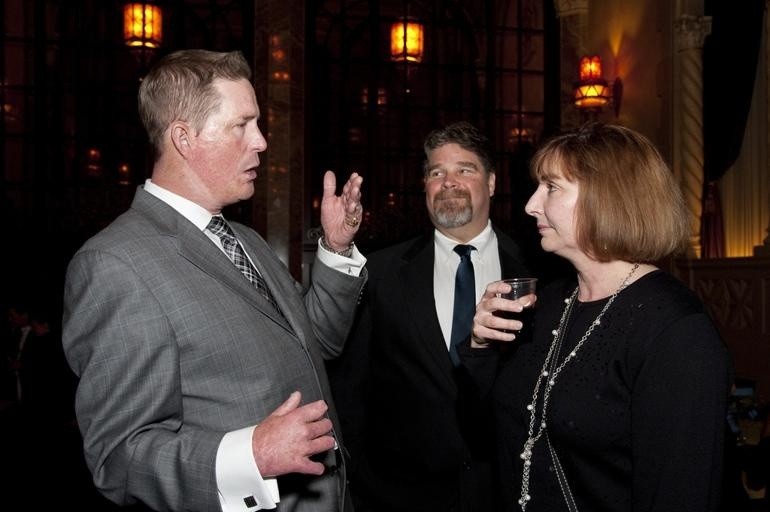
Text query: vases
5 370 21 400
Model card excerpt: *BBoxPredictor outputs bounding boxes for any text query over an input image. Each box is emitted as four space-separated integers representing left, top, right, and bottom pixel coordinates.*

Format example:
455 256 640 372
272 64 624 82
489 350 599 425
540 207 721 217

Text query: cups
502 278 536 331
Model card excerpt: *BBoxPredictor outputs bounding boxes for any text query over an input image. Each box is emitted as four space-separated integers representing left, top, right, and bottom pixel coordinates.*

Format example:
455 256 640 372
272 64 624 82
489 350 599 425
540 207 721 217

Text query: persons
456 133 729 511
344 127 562 511
62 50 369 512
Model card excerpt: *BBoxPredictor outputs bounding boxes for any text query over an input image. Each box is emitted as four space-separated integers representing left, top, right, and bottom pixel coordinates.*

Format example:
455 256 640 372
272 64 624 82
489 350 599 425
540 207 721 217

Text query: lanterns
576 53 610 105
124 15 163 46
390 19 426 62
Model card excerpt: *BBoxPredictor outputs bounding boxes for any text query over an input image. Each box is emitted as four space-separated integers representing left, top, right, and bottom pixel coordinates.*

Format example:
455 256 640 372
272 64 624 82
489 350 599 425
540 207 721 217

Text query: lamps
389 1 423 67
572 52 622 117
124 4 162 48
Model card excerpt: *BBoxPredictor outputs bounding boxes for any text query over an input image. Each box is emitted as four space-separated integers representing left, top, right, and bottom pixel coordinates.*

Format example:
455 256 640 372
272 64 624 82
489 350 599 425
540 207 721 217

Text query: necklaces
519 260 638 512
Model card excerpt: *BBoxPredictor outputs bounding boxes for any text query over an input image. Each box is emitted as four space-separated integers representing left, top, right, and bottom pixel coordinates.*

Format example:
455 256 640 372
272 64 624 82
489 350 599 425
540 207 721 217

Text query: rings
345 214 360 228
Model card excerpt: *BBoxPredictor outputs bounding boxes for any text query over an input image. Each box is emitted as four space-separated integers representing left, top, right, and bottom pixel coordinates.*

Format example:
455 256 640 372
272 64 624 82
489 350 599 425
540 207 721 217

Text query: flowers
0 309 50 376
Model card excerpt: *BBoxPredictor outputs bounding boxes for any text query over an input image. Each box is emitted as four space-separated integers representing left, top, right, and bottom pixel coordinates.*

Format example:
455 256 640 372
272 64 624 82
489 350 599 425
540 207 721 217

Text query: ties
449 244 477 375
206 217 287 321
